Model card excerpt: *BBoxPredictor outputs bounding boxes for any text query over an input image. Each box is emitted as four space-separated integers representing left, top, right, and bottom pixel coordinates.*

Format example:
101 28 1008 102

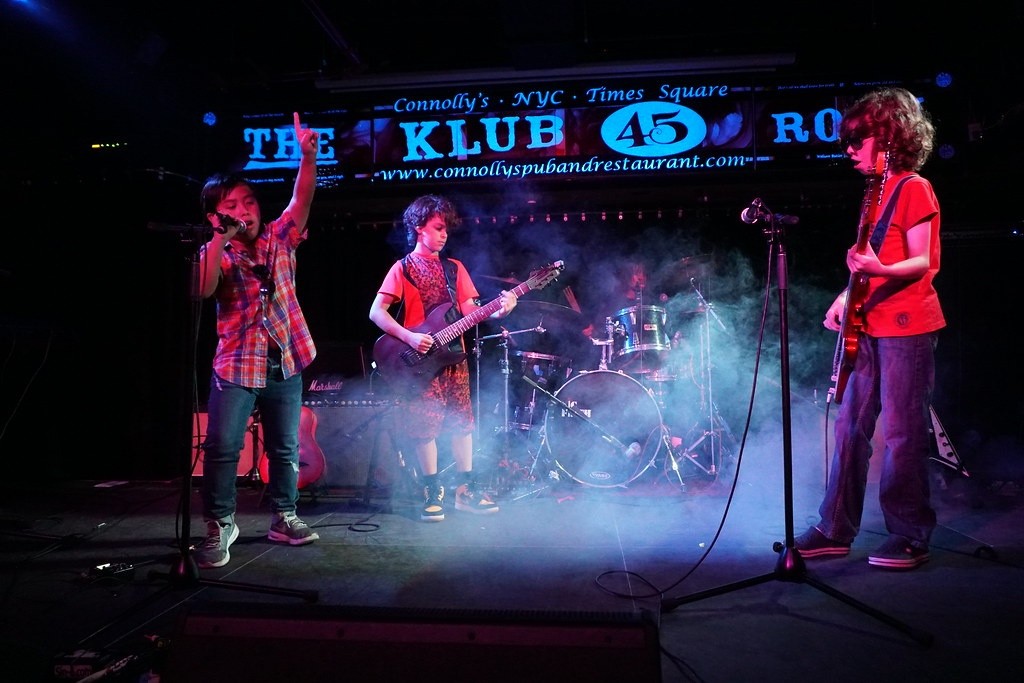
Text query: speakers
159 598 664 683
298 393 401 487
189 403 268 486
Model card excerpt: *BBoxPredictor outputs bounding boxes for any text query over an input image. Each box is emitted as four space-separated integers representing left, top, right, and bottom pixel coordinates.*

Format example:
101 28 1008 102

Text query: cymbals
487 300 590 333
661 255 711 286
478 273 522 286
684 308 719 314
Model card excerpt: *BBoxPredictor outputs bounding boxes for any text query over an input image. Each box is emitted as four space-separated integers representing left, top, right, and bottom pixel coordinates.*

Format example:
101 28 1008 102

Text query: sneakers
869 537 930 568
783 526 851 558
197 520 240 568
421 485 445 521
454 484 500 515
267 512 319 545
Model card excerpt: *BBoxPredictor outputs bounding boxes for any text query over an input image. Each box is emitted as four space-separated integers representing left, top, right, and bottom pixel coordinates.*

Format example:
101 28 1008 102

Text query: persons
782 88 947 569
370 196 516 522
195 112 320 568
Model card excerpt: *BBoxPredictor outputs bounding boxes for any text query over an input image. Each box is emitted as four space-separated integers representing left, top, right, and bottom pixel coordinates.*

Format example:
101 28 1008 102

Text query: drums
644 373 678 414
544 369 664 489
495 348 573 434
608 306 672 370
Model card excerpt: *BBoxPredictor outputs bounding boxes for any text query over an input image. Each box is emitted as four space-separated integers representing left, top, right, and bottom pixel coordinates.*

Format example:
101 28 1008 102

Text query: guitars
832 165 877 405
371 261 564 404
259 405 327 488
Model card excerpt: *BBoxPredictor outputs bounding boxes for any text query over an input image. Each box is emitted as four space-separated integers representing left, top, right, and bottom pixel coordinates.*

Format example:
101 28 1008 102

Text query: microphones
216 212 246 232
625 442 641 457
633 282 642 302
741 198 763 225
343 433 362 441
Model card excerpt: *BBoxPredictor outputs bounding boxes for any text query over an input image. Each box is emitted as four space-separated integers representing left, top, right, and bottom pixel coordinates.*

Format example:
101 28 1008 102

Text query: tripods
60 221 319 658
470 329 559 486
657 211 937 652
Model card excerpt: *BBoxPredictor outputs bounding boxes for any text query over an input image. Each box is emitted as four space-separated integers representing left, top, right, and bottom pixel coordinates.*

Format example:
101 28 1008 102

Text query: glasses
840 133 877 157
